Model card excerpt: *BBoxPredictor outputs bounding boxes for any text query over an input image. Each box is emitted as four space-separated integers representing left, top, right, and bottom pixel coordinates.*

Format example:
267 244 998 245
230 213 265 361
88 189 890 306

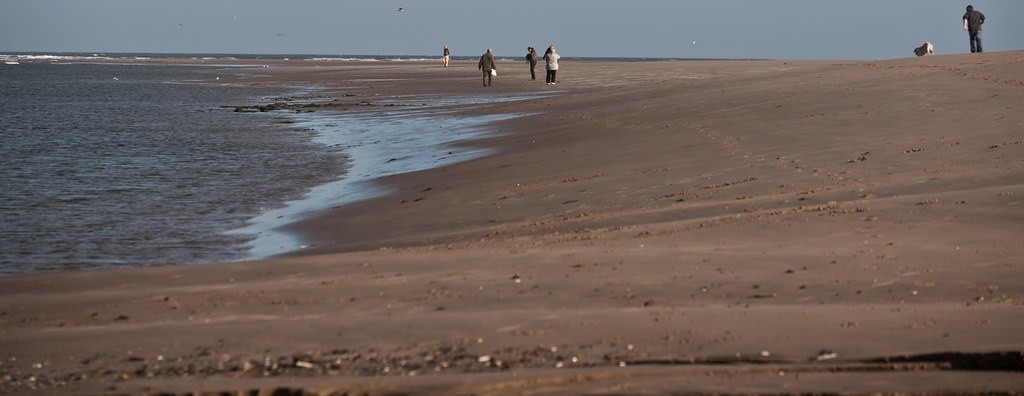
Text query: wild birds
689 38 699 45
396 6 406 13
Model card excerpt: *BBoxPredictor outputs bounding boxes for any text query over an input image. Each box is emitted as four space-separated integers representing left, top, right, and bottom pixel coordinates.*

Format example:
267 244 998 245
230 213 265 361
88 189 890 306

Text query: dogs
913 42 935 57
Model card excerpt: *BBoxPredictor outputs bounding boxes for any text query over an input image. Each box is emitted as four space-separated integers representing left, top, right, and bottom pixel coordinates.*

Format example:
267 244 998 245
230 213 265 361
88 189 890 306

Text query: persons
478 49 496 87
443 45 450 67
526 46 537 81
542 44 560 85
963 5 985 53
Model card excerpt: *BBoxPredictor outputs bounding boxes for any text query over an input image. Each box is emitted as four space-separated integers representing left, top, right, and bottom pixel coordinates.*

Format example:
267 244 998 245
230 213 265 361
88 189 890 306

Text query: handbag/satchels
963 18 969 30
491 68 497 77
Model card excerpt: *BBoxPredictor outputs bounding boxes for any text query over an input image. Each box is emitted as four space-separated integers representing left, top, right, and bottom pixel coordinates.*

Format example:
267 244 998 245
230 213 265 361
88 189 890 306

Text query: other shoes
547 83 550 85
551 82 555 85
